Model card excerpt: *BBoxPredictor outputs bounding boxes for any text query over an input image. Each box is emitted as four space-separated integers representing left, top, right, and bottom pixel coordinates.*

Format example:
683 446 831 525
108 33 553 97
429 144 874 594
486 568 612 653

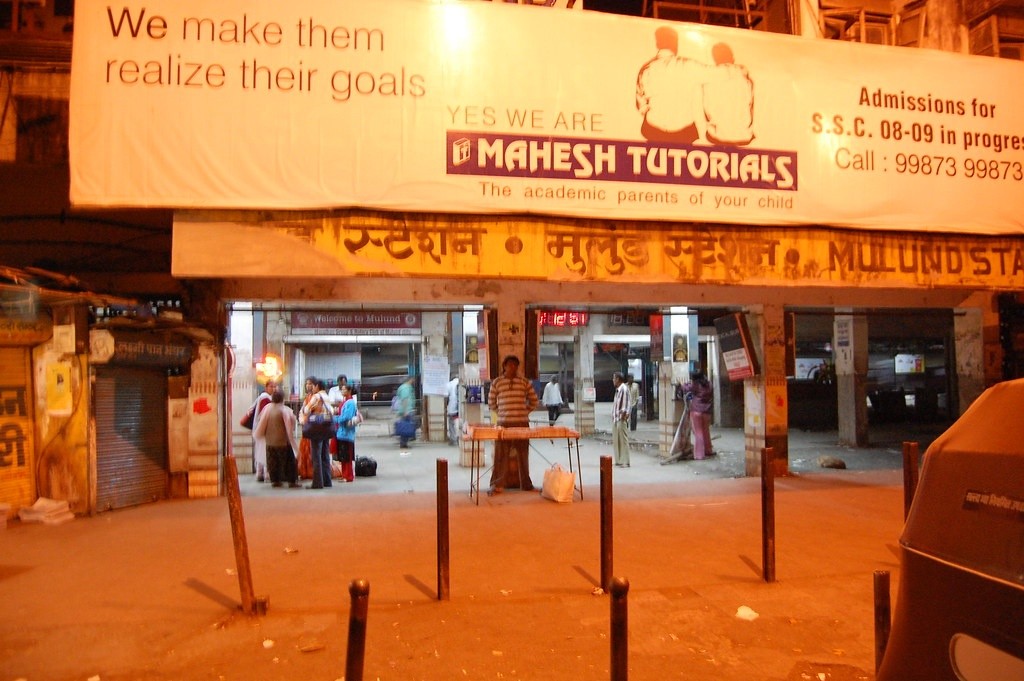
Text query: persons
488 355 541 496
636 25 756 148
612 371 639 470
679 370 718 461
240 375 363 491
391 374 416 450
444 372 459 446
541 375 563 447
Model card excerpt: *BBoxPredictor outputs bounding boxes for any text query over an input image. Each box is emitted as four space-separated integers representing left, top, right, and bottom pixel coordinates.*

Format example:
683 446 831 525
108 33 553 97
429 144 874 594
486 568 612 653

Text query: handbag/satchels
346 413 363 427
390 395 400 410
301 392 336 440
541 462 576 504
394 415 416 437
355 454 378 476
240 403 257 429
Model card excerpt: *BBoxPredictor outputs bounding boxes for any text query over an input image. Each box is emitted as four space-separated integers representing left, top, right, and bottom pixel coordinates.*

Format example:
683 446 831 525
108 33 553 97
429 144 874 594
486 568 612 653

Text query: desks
462 429 583 505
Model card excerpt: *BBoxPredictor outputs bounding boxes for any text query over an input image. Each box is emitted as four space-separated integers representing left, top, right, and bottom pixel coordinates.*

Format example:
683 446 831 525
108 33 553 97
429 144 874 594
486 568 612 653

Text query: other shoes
306 485 323 489
487 486 504 495
531 487 540 492
272 481 284 486
400 448 412 455
289 480 303 488
615 463 630 467
256 475 271 483
339 477 348 481
